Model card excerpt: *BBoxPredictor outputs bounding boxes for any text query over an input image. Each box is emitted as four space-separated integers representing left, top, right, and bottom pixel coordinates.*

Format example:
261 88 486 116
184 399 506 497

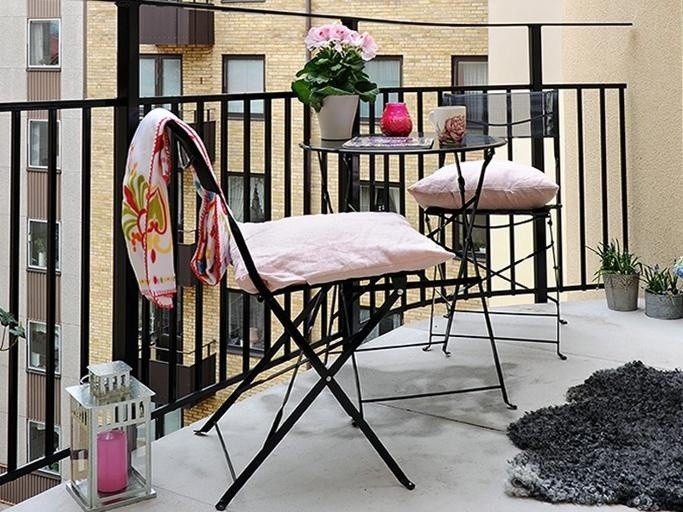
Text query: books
342 137 434 150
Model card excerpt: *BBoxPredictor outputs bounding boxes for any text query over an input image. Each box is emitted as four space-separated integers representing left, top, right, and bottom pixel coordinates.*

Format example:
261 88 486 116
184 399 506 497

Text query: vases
314 95 360 141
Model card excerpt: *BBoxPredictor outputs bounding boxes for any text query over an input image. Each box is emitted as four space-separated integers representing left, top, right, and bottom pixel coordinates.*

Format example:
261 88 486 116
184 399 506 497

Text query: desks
302 134 517 424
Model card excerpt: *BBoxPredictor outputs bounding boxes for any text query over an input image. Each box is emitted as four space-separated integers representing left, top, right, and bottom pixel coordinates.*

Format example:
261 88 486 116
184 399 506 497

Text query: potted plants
585 239 683 320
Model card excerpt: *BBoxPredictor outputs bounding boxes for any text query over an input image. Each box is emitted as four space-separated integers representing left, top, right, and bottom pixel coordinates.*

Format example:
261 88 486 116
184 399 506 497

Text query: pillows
222 209 456 297
411 160 557 209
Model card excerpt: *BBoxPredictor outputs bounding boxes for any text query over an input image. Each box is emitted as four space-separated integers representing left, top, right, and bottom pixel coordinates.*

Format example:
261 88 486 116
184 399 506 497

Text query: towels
119 107 228 311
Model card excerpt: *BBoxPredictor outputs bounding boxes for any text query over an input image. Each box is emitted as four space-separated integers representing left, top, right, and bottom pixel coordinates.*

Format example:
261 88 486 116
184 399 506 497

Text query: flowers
293 21 379 113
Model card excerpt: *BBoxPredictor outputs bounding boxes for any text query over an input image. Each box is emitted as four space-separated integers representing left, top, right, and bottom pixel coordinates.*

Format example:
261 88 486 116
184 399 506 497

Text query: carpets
506 359 683 512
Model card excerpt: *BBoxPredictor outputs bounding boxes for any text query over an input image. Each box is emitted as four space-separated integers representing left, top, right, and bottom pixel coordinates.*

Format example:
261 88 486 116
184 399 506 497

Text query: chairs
166 120 414 509
408 88 571 361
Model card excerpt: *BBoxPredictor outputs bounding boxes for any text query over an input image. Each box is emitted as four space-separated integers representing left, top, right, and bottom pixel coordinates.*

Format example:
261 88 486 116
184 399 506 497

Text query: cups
429 106 466 148
